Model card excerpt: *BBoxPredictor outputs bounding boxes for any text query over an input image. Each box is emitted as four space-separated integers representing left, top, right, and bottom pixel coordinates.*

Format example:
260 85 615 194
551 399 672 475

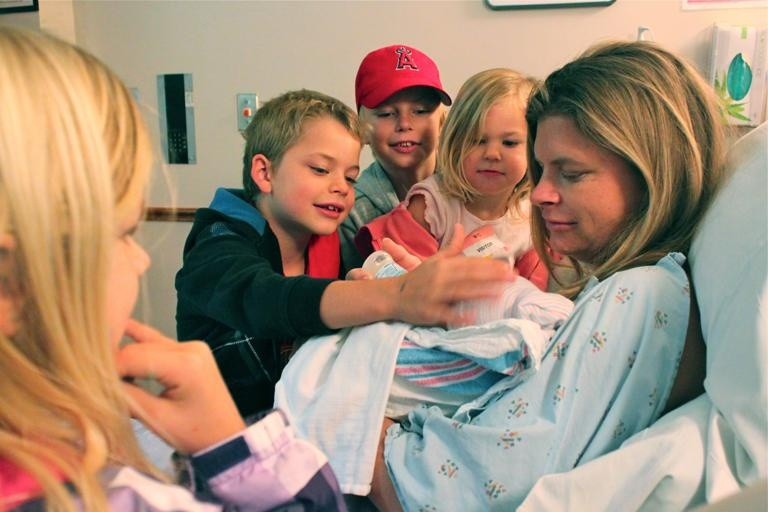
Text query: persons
356 66 563 292
175 87 521 420
0 19 354 512
345 36 732 512
337 43 453 280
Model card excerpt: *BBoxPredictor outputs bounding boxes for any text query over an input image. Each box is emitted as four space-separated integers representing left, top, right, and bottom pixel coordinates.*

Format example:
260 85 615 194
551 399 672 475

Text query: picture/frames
485 0 615 10
0 0 39 13
682 0 768 10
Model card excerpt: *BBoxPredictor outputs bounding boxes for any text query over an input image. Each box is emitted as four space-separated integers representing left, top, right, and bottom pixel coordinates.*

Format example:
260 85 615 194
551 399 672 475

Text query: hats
446 275 577 332
354 46 452 112
